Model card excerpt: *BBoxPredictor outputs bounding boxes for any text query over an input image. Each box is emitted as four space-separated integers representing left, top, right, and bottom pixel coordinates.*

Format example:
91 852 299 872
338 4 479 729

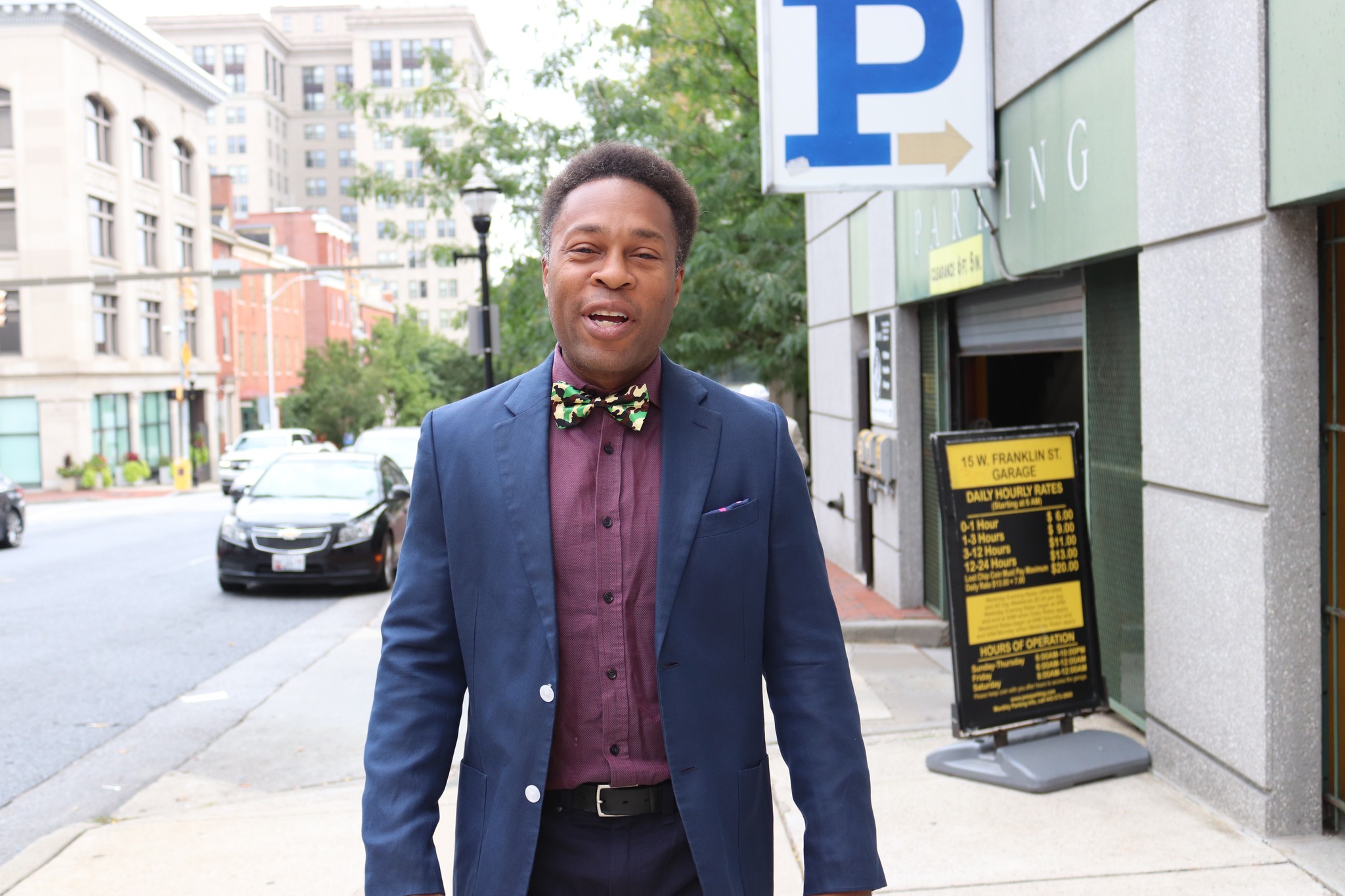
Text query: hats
740 383 770 401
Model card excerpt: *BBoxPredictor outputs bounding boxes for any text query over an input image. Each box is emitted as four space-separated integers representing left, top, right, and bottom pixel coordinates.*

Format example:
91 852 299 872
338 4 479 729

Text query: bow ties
551 380 649 433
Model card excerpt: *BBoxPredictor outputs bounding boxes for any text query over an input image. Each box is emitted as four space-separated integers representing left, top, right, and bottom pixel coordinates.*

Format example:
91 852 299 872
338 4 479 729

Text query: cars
215 452 412 596
0 481 27 552
217 426 423 505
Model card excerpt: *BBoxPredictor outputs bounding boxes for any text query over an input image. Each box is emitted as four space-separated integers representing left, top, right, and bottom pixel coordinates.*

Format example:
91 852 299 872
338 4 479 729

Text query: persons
358 145 891 896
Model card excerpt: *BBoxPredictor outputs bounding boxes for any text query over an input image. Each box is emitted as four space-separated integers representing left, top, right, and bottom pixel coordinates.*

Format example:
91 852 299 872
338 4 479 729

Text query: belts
543 781 677 817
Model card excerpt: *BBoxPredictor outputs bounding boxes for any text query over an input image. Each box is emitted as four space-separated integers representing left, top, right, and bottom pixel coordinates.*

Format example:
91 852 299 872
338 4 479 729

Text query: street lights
457 158 508 395
